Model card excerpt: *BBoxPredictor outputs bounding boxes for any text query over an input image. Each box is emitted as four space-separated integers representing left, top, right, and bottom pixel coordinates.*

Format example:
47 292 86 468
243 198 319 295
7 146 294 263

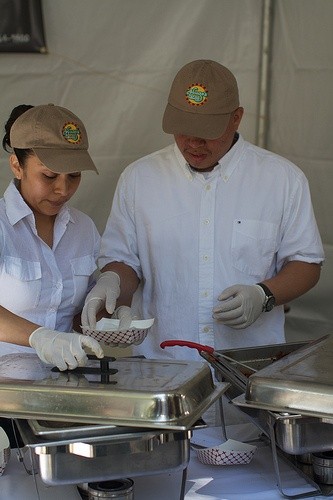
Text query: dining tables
0 424 333 500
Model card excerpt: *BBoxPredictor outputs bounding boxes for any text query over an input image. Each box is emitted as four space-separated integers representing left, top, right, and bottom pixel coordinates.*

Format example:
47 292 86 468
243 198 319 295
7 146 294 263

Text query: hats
10 103 99 174
162 60 239 139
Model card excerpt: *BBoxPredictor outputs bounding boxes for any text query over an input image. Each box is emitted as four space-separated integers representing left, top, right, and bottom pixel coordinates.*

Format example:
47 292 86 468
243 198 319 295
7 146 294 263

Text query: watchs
255 282 276 313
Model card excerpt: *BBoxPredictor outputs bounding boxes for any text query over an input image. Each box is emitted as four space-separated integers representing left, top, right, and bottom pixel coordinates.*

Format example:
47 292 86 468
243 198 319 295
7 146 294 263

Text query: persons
0 103 142 450
80 58 327 430
